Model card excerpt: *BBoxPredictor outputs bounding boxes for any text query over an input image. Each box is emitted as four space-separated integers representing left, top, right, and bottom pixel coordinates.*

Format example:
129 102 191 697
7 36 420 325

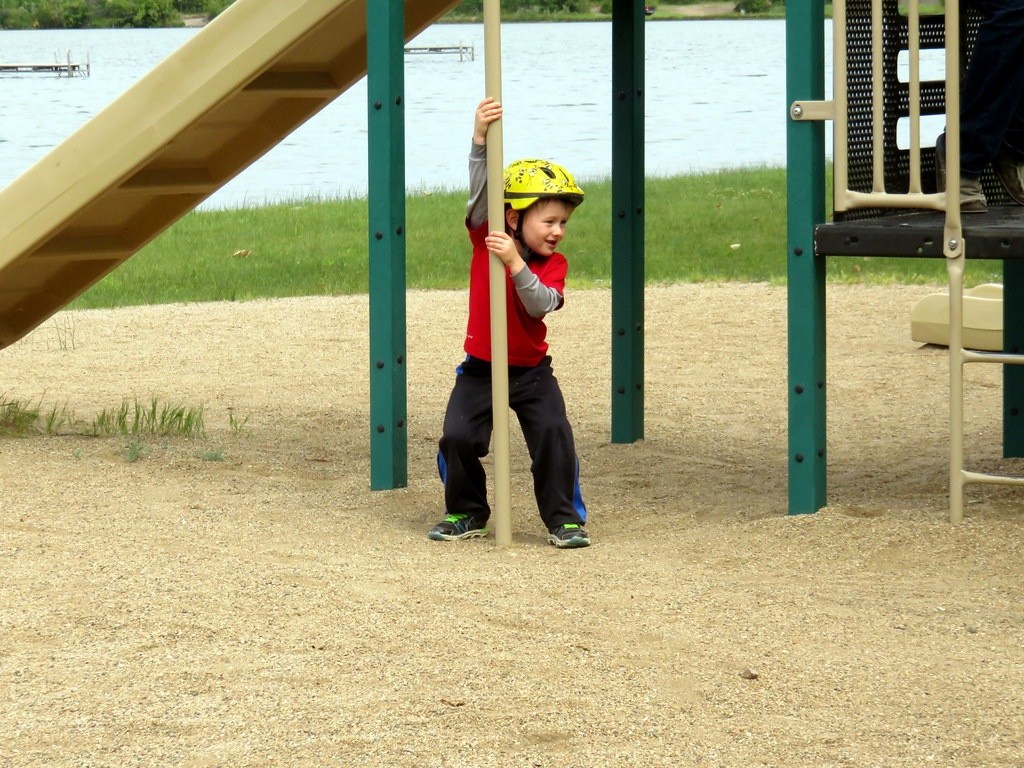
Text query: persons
935 0 1024 212
428 97 590 546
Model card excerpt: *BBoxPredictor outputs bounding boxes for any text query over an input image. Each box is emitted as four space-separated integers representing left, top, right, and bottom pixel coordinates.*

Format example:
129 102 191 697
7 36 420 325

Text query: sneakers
546 523 590 548
428 513 489 541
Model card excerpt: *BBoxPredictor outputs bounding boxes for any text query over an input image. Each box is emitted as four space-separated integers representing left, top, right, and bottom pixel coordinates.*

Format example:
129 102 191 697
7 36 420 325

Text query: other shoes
991 158 1024 204
937 134 988 213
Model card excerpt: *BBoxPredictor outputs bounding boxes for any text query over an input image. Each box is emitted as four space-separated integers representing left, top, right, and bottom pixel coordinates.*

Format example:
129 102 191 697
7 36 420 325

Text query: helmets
504 158 585 210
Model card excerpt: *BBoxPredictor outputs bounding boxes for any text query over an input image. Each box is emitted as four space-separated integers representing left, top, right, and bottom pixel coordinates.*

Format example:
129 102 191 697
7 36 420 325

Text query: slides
0 0 460 355
